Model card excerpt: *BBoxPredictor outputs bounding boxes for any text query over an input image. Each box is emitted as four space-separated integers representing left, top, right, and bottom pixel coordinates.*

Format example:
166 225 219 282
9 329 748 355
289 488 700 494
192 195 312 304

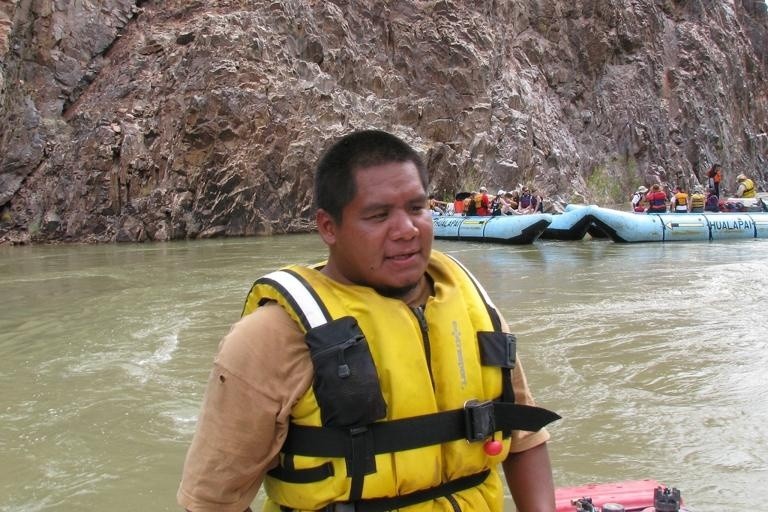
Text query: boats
431 201 598 247
566 197 768 244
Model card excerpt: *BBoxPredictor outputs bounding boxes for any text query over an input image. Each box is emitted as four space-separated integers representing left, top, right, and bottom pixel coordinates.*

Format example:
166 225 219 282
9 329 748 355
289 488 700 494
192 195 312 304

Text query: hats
637 184 663 193
497 190 507 196
480 187 487 192
736 174 747 181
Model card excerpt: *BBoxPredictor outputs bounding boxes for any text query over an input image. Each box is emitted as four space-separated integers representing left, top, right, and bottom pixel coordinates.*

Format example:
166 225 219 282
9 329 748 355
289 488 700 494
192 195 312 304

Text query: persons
629 163 756 214
175 128 557 512
428 187 549 214
